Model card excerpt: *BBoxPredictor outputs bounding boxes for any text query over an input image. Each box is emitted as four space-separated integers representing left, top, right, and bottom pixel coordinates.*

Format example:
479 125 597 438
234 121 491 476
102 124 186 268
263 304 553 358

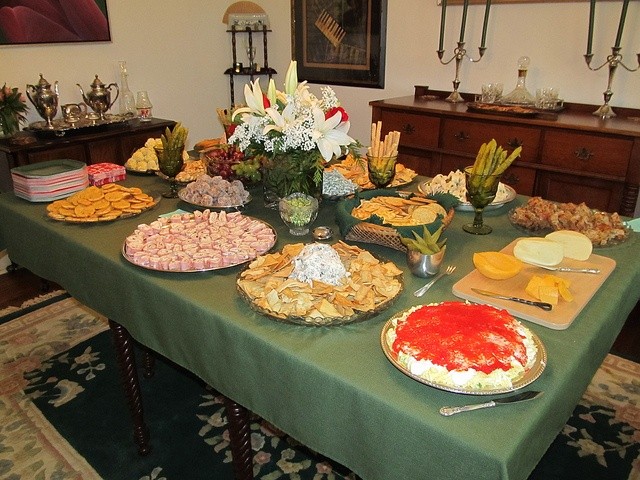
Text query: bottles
118 60 136 117
504 57 535 106
137 91 153 122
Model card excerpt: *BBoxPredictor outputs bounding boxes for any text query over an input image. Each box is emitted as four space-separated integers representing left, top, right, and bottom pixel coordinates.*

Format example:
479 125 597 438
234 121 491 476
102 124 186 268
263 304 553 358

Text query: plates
11 158 90 203
124 162 158 176
154 171 195 186
507 202 634 243
236 241 405 327
45 186 162 223
178 186 253 207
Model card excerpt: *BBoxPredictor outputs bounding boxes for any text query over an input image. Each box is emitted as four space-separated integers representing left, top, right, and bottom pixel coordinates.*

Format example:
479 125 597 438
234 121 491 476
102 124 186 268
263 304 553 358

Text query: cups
535 88 556 109
407 249 446 278
491 83 505 103
475 82 497 105
547 89 561 110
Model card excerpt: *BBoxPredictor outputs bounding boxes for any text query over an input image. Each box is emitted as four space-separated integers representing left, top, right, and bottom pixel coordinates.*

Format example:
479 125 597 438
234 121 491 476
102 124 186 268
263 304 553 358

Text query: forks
413 266 457 298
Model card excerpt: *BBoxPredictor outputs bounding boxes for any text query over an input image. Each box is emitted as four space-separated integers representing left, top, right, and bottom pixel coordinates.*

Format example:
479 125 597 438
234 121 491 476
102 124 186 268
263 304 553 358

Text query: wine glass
366 155 398 188
247 47 256 72
153 142 185 199
462 165 501 234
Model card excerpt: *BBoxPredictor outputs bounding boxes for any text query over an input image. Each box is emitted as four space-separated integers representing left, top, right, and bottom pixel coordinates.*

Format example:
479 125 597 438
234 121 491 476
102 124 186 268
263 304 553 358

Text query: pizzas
386 301 539 390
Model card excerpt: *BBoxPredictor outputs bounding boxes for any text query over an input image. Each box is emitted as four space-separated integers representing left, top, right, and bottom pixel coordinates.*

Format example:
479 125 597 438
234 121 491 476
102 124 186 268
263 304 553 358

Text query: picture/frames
0 0 111 45
291 0 388 88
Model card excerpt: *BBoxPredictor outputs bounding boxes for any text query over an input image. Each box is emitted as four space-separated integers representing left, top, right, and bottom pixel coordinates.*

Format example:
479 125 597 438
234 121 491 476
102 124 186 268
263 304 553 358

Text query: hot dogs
194 138 220 154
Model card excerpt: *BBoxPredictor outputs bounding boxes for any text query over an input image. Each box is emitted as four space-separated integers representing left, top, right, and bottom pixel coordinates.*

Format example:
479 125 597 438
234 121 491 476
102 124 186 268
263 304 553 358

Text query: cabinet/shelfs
369 96 543 198
0 133 89 180
534 112 640 219
89 113 179 174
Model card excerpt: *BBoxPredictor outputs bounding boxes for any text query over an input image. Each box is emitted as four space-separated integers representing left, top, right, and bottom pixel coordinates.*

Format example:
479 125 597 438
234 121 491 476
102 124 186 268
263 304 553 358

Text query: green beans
401 223 448 254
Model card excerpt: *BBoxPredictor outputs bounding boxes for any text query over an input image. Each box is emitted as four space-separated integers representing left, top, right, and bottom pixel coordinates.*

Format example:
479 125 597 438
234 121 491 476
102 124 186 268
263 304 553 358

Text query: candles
584 0 596 65
611 0 629 55
457 0 468 49
478 0 491 56
436 0 447 60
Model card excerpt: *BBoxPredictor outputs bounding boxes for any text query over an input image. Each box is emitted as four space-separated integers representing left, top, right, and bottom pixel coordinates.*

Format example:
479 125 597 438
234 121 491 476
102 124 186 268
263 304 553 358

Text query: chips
238 240 402 318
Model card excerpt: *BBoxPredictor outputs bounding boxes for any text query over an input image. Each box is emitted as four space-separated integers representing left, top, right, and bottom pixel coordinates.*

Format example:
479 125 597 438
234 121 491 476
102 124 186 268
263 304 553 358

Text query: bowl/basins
278 193 319 235
199 146 266 186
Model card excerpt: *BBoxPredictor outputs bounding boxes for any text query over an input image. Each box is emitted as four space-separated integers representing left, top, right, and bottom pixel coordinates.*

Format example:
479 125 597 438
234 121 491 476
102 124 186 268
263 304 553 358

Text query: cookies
323 156 418 189
351 196 447 226
47 183 154 222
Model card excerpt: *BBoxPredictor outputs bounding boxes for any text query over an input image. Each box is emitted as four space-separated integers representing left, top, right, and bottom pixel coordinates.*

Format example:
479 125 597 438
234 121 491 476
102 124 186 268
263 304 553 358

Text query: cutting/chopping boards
452 238 617 332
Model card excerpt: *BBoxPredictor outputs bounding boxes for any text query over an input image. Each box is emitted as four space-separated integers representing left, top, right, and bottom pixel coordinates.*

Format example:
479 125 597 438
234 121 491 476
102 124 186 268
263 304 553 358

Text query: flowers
0 83 31 135
228 60 367 200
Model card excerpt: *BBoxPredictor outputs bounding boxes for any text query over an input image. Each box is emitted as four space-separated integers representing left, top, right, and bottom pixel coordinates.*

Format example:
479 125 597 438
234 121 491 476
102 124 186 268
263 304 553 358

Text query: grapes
206 147 244 182
231 154 262 182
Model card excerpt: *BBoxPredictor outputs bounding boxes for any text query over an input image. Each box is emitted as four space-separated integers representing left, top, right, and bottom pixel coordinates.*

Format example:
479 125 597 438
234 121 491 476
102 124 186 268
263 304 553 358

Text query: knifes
471 288 552 310
530 263 601 274
439 390 546 416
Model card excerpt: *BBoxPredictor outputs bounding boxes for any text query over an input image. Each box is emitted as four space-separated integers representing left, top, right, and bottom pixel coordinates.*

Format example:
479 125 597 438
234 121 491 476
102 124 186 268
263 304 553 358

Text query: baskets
345 191 455 254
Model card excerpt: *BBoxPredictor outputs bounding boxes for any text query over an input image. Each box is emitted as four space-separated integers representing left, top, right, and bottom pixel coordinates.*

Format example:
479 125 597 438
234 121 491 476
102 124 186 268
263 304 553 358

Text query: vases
263 168 323 210
3 123 20 137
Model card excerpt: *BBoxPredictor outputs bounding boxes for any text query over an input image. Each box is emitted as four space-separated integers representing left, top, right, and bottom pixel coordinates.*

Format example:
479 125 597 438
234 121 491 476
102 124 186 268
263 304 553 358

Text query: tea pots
26 72 59 129
61 103 87 123
76 75 120 121
